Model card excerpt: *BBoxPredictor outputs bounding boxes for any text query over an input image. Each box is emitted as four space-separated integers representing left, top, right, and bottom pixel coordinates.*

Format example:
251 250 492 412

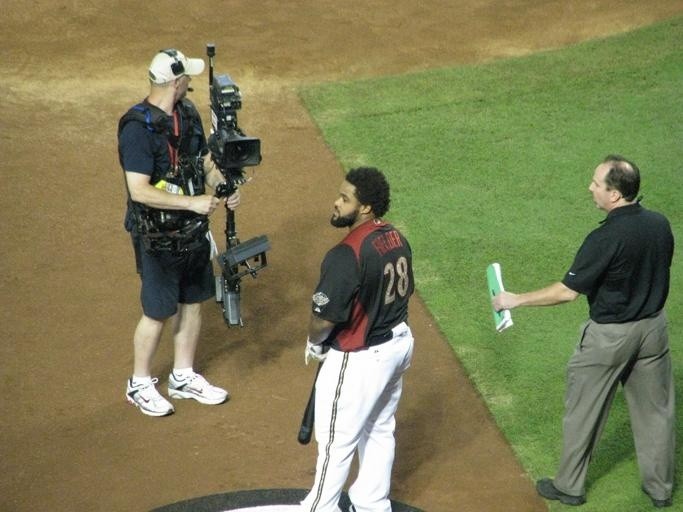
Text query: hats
149 49 205 84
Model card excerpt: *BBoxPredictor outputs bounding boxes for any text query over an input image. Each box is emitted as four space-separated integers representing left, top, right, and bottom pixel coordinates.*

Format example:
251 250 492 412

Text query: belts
367 331 393 346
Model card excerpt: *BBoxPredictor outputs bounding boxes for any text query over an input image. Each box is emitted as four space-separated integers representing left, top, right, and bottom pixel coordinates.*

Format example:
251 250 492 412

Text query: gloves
305 337 325 366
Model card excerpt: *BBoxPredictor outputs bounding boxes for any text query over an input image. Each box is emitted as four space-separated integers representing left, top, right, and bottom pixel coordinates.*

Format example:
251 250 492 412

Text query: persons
491 153 677 510
118 47 242 417
301 165 415 512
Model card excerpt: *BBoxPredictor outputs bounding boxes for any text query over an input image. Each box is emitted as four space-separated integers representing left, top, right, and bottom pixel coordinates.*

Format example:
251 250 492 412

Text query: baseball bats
298 341 331 444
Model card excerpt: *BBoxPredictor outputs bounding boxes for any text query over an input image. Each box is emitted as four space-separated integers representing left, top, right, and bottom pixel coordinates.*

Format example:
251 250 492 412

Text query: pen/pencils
492 289 501 317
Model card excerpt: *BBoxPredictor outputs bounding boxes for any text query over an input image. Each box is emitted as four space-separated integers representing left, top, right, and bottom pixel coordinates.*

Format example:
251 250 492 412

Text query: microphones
188 88 193 91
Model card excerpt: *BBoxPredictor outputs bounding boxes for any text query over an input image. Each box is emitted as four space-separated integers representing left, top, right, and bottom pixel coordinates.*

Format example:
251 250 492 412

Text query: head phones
160 49 185 75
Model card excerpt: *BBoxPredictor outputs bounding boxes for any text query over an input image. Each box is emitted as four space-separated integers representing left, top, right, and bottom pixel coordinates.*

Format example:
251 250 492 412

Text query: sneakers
168 372 229 404
537 479 586 505
641 486 671 507
126 378 175 417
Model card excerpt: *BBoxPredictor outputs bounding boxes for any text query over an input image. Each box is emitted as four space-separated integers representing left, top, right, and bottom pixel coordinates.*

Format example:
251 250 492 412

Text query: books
485 263 514 334
